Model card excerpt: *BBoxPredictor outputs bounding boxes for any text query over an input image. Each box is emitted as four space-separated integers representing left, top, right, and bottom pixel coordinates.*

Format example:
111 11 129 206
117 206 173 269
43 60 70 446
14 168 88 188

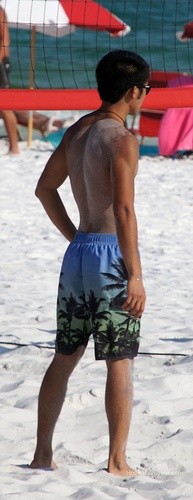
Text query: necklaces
94 110 136 135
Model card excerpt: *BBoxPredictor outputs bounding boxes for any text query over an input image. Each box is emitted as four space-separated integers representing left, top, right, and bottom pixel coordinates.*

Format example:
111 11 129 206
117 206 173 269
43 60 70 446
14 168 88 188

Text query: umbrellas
0 0 131 146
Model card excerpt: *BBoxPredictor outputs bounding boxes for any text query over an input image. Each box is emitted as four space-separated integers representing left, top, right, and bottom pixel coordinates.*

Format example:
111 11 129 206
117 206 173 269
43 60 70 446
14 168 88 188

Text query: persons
0 3 19 156
28 49 152 476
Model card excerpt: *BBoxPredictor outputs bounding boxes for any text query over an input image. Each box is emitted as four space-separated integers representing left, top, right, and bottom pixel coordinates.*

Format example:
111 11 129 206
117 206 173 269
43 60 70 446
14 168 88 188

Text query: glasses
137 85 151 95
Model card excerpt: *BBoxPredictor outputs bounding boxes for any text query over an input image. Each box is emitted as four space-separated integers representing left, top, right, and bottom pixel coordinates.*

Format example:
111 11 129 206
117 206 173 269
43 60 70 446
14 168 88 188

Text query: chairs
129 81 171 145
